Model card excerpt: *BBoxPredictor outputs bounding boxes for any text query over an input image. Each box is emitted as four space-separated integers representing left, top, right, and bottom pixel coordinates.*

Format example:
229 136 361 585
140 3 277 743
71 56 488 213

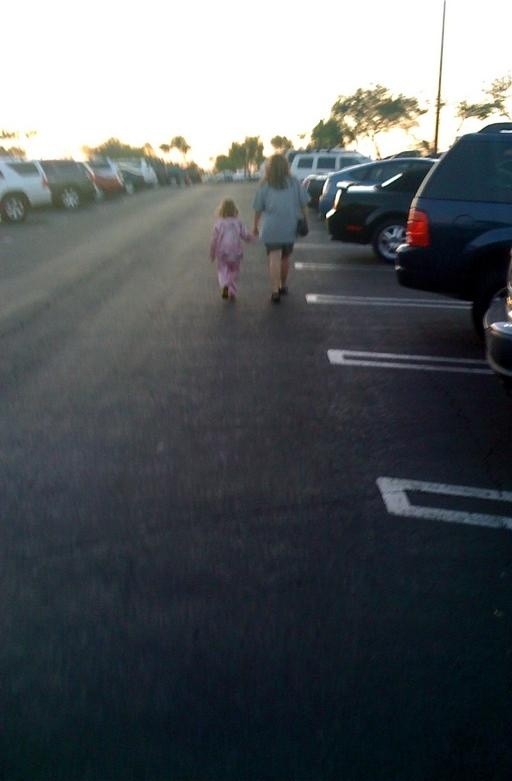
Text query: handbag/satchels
297 218 308 236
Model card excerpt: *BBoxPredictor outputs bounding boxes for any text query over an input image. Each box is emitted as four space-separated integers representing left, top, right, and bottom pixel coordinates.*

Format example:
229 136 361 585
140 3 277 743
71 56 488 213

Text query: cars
214 171 261 182
0 156 190 224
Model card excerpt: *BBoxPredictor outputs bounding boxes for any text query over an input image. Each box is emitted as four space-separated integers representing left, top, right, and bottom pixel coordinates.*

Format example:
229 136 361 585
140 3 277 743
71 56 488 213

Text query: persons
208 199 256 301
251 154 312 305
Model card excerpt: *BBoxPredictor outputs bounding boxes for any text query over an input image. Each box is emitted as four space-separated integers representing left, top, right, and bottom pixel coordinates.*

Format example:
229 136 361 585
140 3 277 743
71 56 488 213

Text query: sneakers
222 286 236 302
272 286 288 301
